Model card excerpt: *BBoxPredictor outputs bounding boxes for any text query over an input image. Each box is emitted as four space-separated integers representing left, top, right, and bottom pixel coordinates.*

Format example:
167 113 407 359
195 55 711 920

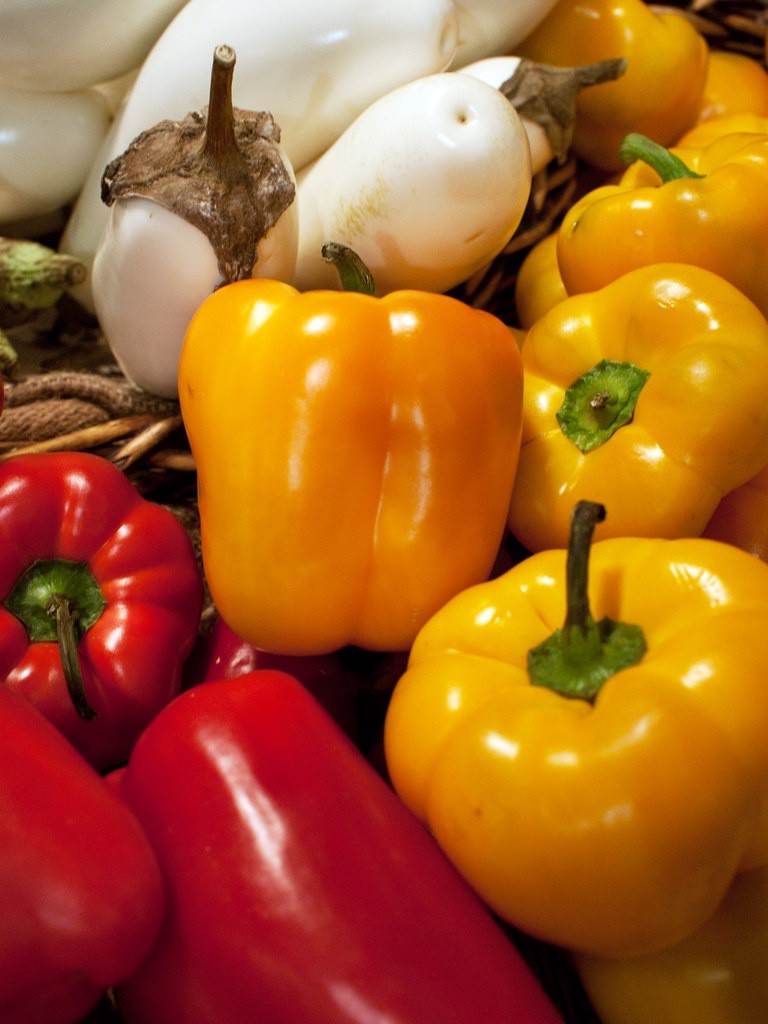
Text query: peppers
0 241 768 1024
509 0 767 331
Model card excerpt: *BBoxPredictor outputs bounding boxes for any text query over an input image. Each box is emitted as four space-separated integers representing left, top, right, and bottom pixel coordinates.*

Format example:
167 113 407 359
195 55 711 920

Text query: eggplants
0 0 539 396
